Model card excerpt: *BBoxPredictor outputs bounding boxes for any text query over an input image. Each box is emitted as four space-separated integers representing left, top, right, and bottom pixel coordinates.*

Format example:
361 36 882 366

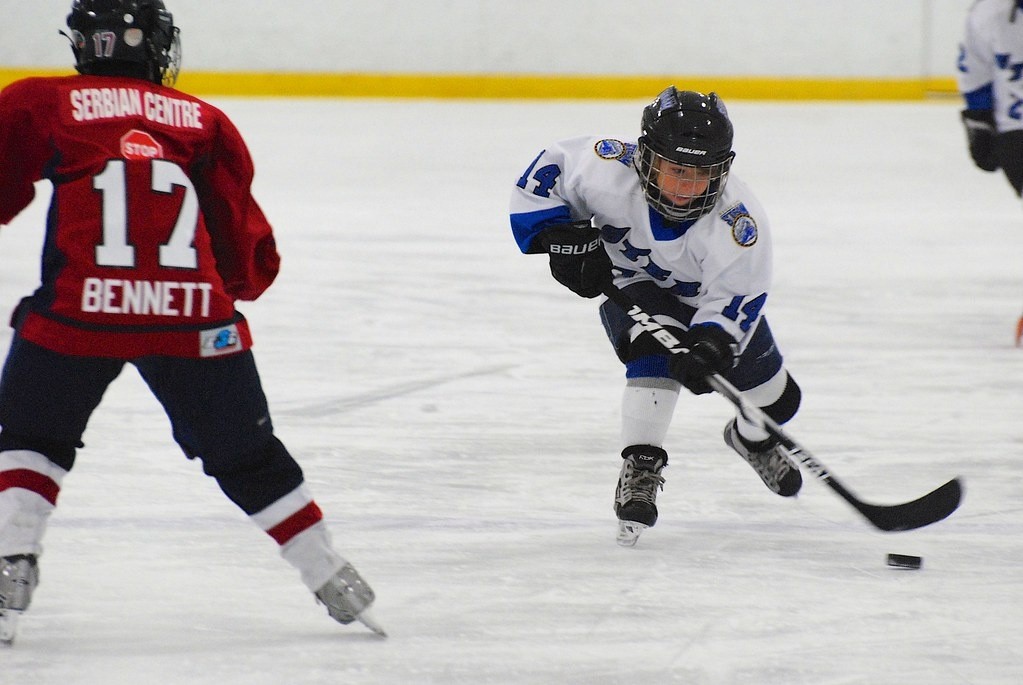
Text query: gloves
537 220 614 299
674 324 734 395
962 108 1001 172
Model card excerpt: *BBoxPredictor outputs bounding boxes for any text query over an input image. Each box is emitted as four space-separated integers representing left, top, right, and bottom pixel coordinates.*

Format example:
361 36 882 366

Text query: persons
958 1 1023 204
2 1 376 640
503 85 802 530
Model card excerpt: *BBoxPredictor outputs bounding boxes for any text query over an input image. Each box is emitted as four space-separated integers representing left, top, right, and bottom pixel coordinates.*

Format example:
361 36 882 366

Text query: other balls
886 553 923 569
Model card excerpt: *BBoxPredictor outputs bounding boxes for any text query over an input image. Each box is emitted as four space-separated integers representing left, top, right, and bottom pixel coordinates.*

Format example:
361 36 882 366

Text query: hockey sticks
602 283 967 536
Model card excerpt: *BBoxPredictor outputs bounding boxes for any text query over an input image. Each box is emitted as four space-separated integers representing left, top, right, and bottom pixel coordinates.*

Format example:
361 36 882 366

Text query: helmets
637 86 736 224
59 0 182 86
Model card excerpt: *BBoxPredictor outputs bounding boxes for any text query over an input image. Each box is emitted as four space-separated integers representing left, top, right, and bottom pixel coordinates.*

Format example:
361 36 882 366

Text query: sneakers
615 444 669 547
724 415 804 500
0 553 39 643
313 560 386 636
